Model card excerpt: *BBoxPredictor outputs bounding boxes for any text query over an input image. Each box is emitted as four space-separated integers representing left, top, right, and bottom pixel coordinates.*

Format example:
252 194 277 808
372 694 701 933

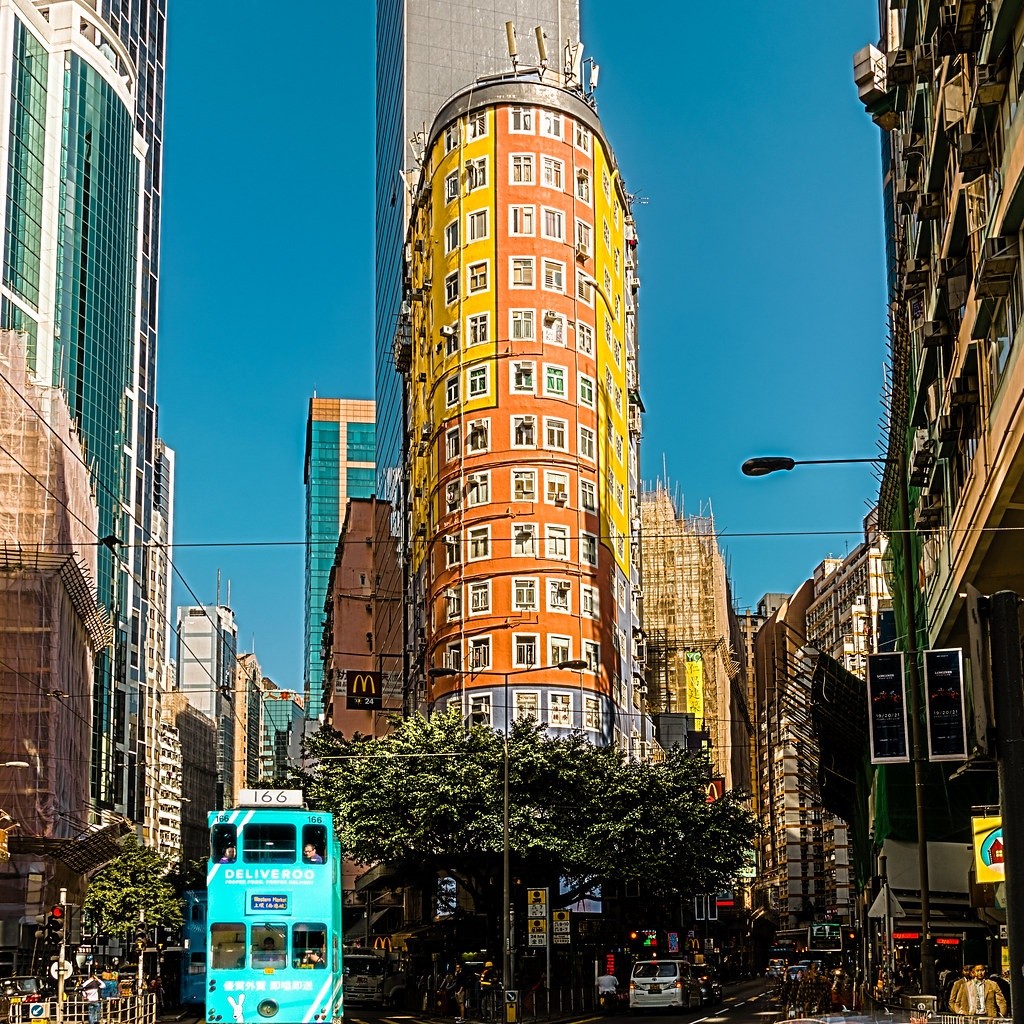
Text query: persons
889 958 1008 1024
220 844 325 968
414 962 494 1024
595 969 619 1009
81 973 106 1024
785 963 850 1016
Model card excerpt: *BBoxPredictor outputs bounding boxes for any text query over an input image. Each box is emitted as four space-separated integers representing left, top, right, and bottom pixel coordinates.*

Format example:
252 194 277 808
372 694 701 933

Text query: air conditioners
406 644 414 652
520 360 533 371
403 180 432 606
467 474 481 484
621 212 642 437
439 324 455 338
523 415 533 426
441 589 454 599
522 523 533 534
556 491 568 501
473 419 488 430
464 158 475 171
579 168 589 180
545 310 558 323
558 581 572 591
906 428 930 490
418 638 427 645
441 534 452 545
576 242 590 259
912 494 941 528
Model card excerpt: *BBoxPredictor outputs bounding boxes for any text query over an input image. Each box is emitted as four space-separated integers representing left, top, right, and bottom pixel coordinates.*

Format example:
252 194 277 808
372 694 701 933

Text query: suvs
0 973 136 1018
691 964 723 1008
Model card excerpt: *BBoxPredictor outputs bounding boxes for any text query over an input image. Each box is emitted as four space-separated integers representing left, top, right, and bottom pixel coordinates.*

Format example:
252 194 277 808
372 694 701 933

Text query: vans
629 960 703 1013
765 959 822 979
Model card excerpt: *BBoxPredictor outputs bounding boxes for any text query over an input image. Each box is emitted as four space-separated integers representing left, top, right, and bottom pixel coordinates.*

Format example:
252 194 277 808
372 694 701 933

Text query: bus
206 789 344 1024
180 889 207 1004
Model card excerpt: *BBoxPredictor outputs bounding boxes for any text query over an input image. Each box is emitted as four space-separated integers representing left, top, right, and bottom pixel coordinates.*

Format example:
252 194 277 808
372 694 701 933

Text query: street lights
428 660 588 989
742 453 932 996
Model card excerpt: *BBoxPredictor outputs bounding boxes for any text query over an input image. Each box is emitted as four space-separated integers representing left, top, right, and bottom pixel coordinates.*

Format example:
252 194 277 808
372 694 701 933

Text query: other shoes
454 1017 466 1024
482 1016 490 1019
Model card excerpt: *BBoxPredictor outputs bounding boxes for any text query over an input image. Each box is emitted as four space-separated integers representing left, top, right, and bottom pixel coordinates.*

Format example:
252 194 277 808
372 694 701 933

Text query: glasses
304 848 314 853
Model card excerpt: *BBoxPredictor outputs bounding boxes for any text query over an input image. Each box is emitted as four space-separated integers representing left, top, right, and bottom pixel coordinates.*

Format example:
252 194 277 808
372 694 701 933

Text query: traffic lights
708 894 718 920
695 894 705 920
135 921 146 952
35 913 52 944
50 904 65 944
157 943 165 963
629 932 648 942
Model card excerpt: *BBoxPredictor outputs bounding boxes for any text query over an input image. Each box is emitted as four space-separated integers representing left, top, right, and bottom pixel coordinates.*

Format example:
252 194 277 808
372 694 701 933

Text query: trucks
344 947 387 1009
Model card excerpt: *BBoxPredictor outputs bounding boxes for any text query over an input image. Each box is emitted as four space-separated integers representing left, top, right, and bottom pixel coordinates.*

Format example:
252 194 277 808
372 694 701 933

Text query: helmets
485 961 493 967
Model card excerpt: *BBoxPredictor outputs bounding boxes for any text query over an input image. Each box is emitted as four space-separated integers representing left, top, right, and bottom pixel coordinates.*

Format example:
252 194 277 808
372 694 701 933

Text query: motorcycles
601 991 617 1015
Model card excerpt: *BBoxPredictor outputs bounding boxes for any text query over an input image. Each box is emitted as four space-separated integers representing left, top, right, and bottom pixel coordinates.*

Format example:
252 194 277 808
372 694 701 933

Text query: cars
783 966 808 983
